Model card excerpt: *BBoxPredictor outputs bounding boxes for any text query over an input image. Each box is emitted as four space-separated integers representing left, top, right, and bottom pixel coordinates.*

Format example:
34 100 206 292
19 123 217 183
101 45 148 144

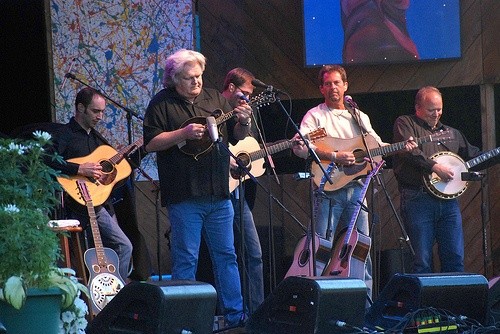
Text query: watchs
238 117 251 126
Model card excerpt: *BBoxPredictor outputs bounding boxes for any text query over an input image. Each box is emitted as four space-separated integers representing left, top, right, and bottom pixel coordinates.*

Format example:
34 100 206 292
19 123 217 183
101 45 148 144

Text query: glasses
230 82 252 98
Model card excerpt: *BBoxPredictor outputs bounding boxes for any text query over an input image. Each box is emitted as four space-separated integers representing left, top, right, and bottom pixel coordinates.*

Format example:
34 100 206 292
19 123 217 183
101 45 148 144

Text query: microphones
206 116 219 152
344 95 359 110
236 91 251 104
251 79 287 95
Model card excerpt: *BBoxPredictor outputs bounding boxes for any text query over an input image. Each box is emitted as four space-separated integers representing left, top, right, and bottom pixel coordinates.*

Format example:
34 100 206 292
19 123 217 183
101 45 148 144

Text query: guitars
322 159 387 282
176 85 276 162
420 146 500 201
307 126 458 192
74 177 128 319
284 162 337 279
227 127 328 194
53 137 144 216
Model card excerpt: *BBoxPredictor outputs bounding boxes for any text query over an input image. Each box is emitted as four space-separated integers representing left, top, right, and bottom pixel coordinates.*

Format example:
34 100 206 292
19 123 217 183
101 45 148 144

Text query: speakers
84 280 218 334
246 274 500 334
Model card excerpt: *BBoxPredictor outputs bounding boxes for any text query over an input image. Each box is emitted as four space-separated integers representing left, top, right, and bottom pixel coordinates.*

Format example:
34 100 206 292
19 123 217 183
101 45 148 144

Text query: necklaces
328 105 346 119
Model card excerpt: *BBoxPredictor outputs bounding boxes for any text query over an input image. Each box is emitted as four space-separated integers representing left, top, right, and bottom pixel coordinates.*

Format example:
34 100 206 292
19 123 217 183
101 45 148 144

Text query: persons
41 88 148 283
394 87 500 273
293 64 418 315
221 68 310 316
143 49 254 330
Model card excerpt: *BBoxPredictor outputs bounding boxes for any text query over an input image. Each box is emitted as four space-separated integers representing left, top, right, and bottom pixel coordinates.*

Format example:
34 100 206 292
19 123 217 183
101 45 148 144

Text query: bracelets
331 152 336 162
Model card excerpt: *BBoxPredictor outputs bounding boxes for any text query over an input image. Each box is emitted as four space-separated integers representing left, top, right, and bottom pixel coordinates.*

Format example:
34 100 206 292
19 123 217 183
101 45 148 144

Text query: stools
48 219 85 282
